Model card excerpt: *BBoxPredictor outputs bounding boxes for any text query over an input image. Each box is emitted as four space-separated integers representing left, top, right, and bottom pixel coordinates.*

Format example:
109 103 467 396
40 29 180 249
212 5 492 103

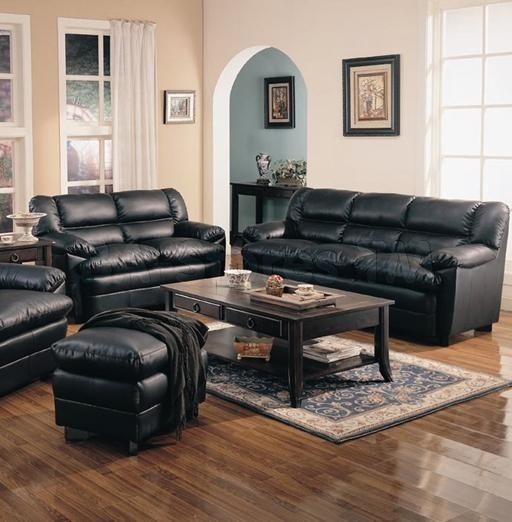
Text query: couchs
239 182 511 346
28 183 231 328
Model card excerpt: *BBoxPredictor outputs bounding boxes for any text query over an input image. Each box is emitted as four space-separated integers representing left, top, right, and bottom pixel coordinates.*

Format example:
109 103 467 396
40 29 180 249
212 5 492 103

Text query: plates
294 289 315 295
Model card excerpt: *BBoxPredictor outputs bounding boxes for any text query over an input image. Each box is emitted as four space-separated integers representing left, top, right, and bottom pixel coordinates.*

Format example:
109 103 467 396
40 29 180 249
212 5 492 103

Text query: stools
49 317 209 458
0 260 75 402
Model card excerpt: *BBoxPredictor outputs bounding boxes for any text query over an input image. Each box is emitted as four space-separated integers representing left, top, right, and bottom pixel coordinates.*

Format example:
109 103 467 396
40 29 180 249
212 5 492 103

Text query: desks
0 241 56 268
231 180 296 242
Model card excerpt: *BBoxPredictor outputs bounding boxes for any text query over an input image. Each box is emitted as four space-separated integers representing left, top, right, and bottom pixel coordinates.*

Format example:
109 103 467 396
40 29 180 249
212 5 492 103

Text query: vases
279 177 304 189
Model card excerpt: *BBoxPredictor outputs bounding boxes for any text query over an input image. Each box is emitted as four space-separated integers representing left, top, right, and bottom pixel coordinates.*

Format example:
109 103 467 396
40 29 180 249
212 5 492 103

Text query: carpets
203 330 511 449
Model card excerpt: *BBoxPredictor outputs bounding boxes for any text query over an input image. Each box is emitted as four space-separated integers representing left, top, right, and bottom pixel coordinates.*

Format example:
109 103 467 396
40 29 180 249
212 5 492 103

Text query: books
301 334 362 364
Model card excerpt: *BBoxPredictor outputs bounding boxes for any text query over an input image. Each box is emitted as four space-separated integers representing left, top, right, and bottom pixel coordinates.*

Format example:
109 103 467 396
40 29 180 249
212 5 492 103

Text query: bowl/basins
298 284 313 291
223 270 252 288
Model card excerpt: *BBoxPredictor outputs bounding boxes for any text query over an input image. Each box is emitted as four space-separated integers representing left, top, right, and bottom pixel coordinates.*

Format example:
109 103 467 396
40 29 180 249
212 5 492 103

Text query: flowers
270 156 306 181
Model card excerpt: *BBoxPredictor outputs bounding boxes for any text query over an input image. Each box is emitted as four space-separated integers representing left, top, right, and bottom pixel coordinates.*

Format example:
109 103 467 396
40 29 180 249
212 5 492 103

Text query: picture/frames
164 88 197 125
264 74 296 132
339 53 401 139
